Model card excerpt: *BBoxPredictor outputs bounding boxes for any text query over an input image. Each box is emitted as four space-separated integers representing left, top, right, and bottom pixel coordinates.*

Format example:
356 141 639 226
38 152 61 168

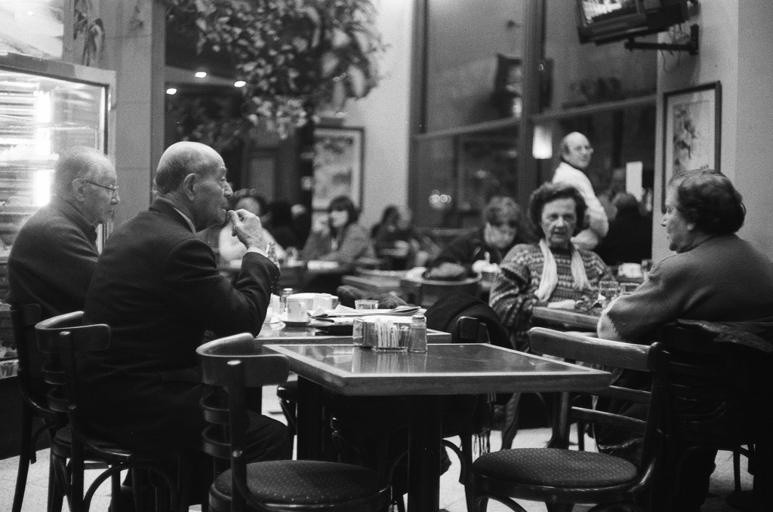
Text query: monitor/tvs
576 0 688 47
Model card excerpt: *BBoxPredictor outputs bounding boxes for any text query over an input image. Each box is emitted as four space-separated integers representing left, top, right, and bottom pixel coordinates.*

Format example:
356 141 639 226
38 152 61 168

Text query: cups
353 299 379 310
598 282 640 307
284 247 296 268
351 318 399 348
287 297 307 321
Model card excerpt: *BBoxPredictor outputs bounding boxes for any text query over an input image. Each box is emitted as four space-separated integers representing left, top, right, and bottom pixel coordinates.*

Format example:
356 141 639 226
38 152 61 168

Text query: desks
261 337 611 494
231 310 454 465
534 298 668 451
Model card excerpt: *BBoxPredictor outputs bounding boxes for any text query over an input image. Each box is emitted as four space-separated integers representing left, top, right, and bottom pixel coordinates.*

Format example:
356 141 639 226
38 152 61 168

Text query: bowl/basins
287 292 338 313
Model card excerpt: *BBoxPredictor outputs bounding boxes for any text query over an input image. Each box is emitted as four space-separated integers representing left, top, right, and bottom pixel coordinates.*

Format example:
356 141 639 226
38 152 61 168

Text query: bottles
410 313 428 354
282 288 292 310
398 325 409 347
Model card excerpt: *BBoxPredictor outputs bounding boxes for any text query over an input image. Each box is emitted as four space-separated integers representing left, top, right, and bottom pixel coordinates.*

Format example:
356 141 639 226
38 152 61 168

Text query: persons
489 181 620 355
608 191 652 268
7 146 191 512
425 194 539 300
596 170 773 512
301 198 379 307
369 206 425 271
72 140 292 462
552 131 608 268
220 195 288 265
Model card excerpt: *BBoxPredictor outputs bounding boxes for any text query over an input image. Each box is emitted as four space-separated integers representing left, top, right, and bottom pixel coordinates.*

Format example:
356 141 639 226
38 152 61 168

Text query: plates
280 317 310 326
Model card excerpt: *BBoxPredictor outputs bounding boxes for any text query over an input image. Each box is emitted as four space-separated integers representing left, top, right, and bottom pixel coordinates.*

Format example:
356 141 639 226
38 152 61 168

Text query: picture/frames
658 80 721 216
311 125 363 213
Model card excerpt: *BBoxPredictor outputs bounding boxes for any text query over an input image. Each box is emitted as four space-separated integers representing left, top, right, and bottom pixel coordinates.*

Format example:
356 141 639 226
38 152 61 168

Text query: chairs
195 331 402 509
251 239 508 327
464 325 663 507
446 292 589 440
670 314 771 509
10 308 173 507
